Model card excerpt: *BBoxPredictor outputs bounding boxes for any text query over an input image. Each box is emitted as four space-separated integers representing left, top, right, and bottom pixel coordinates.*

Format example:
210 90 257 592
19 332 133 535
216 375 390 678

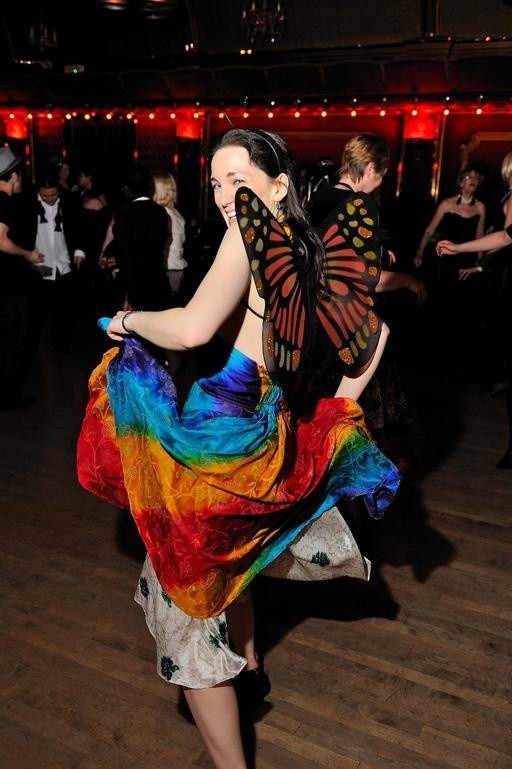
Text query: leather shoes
234 668 271 706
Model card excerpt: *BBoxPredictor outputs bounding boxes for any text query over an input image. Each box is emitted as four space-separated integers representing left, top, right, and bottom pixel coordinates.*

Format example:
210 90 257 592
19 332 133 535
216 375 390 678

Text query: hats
0 148 20 175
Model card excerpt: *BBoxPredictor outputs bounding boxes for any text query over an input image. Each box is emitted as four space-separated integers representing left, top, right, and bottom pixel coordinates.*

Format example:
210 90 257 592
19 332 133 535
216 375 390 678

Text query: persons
82 128 392 769
0 131 512 471
